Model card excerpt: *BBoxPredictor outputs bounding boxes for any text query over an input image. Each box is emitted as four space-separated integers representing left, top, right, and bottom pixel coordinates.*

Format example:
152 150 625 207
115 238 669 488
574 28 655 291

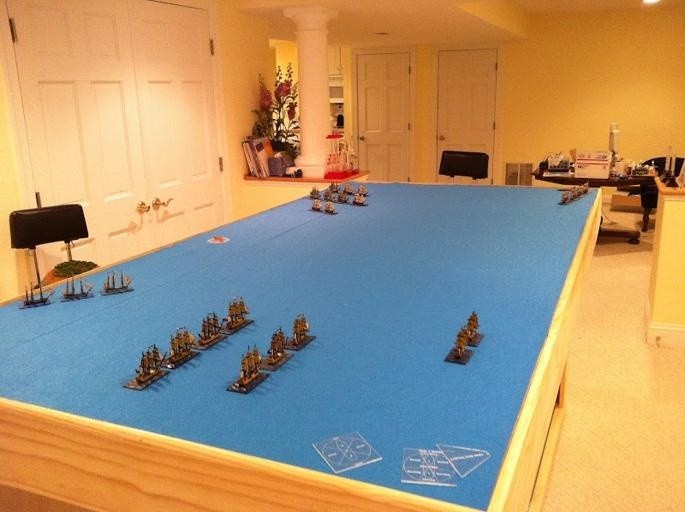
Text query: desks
534 170 655 251
0 183 603 512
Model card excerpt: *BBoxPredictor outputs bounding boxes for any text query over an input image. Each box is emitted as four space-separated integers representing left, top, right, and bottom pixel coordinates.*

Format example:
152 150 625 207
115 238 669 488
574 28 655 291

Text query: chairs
6 204 99 289
439 150 489 185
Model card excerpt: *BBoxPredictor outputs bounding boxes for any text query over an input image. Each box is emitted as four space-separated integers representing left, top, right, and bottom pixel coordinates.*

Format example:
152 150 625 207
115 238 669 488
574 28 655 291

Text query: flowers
246 62 301 156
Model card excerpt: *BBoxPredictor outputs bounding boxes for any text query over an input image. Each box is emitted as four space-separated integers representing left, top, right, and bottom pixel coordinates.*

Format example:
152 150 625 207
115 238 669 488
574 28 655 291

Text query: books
242 134 273 178
